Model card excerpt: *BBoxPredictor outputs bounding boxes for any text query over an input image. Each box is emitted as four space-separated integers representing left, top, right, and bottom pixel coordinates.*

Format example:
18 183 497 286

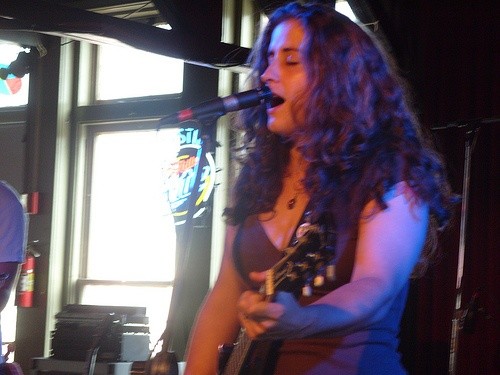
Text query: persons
183 2 453 375
0 180 25 311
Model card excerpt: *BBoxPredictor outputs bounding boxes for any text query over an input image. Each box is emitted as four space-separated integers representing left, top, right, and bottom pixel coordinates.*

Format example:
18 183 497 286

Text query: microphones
429 114 500 131
154 85 271 128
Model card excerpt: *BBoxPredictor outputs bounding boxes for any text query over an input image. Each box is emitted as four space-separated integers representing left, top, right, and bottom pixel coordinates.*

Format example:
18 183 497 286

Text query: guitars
216 204 337 375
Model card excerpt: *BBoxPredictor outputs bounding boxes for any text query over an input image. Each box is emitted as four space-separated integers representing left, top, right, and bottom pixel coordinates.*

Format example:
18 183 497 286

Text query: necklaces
283 180 305 209
